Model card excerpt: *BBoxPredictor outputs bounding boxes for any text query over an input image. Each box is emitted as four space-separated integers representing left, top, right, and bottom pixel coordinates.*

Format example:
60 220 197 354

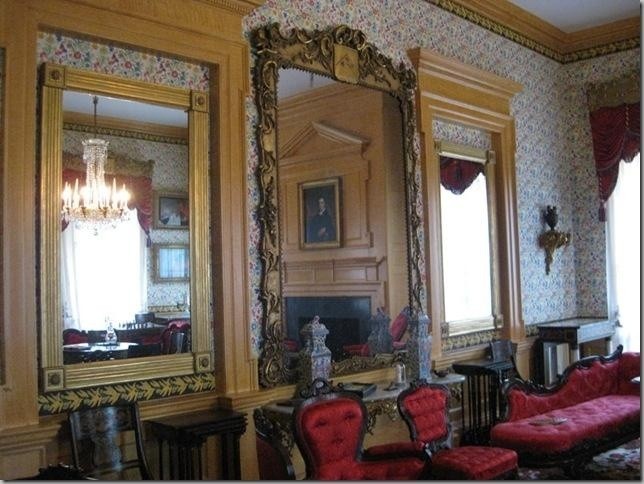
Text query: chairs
68 401 154 480
344 306 409 357
289 376 517 480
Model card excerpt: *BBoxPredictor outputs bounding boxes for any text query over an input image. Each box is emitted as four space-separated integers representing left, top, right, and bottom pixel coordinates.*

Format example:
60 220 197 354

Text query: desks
145 408 247 479
535 317 616 389
62 341 138 364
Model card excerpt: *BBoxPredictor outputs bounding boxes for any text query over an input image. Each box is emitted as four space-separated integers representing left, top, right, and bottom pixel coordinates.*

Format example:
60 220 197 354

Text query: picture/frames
297 176 341 250
150 188 191 283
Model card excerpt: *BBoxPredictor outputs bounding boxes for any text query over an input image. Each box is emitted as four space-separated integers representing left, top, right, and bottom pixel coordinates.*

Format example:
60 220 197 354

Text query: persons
307 195 336 242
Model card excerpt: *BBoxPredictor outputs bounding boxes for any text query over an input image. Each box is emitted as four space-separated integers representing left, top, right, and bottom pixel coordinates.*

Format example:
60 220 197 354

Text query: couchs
63 319 190 357
488 343 641 479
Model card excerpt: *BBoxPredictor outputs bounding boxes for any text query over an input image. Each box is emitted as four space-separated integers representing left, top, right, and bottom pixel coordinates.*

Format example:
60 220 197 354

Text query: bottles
104 326 118 343
395 352 406 389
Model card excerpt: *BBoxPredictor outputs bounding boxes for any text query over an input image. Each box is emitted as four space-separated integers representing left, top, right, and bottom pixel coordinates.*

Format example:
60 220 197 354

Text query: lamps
58 95 128 232
538 204 572 276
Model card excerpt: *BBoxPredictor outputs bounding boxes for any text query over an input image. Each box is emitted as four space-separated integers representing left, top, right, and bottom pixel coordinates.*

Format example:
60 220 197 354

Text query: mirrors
434 140 505 340
35 63 211 393
251 21 425 391
